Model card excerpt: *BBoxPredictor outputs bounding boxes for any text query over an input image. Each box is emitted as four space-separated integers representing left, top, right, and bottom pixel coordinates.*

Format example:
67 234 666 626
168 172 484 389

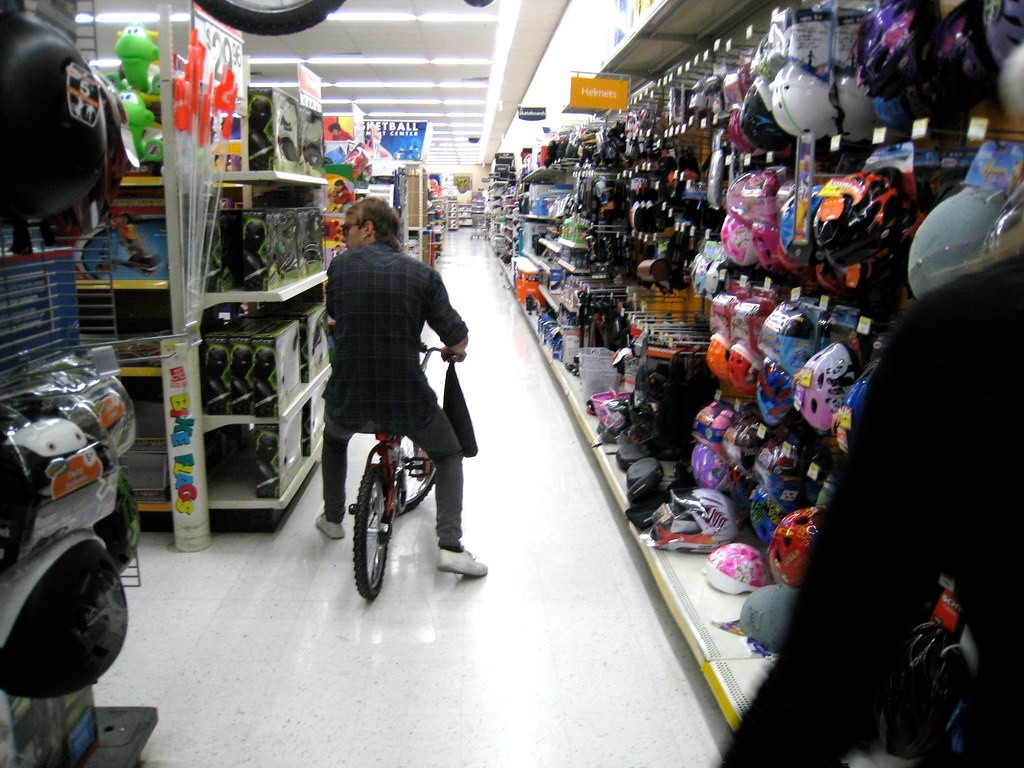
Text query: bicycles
347 340 460 601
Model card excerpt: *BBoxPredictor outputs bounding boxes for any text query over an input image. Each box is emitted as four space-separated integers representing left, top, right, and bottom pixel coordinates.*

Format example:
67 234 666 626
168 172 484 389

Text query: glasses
341 222 363 237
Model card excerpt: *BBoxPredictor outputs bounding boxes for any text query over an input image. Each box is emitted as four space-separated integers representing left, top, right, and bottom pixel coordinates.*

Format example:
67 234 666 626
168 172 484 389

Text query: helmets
0 0 141 258
594 398 634 443
652 0 1024 751
0 531 127 699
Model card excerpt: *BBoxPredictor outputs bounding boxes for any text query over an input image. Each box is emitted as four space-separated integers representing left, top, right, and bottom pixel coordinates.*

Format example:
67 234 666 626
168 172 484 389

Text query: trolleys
470 215 489 240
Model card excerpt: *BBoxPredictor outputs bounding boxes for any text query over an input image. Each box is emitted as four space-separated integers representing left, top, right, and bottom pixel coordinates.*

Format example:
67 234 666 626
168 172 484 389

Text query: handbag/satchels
443 364 480 457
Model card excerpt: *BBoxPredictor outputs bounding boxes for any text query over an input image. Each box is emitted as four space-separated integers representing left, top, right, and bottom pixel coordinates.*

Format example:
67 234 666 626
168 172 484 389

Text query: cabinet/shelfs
187 172 333 534
366 127 578 346
53 93 176 523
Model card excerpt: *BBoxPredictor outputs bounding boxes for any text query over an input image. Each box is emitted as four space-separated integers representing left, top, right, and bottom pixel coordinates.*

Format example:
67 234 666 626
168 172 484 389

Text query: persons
316 196 488 578
328 179 354 213
110 212 151 261
718 253 1024 768
328 122 353 141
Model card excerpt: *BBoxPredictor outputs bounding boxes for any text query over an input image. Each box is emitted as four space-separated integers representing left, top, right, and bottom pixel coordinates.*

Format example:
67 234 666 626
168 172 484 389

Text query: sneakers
439 549 486 576
316 512 345 539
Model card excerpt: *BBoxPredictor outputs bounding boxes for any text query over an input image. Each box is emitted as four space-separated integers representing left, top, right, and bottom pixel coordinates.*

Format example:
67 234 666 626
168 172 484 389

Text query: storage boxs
194 63 327 499
